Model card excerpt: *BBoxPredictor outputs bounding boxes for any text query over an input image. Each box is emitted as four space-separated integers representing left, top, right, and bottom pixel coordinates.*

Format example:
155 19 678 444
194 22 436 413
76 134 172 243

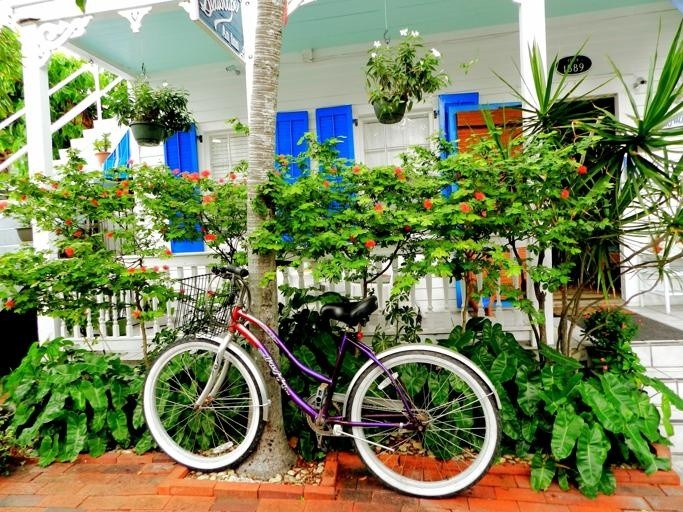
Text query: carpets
554 303 683 341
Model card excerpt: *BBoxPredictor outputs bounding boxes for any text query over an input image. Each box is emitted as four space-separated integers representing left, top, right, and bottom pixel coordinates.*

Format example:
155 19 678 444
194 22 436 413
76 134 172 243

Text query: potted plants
99 73 200 147
0 24 128 174
10 200 32 241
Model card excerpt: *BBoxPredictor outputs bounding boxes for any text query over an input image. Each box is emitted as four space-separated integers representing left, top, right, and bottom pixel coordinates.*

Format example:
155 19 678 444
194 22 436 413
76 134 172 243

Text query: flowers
362 26 483 121
579 303 645 348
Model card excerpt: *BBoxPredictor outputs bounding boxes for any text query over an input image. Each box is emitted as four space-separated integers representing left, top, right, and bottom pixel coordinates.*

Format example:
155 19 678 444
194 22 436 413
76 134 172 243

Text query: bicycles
142 266 502 498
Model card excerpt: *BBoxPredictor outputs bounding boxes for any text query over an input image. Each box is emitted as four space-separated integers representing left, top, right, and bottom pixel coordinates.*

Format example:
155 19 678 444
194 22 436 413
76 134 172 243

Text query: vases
586 345 616 366
370 94 409 126
